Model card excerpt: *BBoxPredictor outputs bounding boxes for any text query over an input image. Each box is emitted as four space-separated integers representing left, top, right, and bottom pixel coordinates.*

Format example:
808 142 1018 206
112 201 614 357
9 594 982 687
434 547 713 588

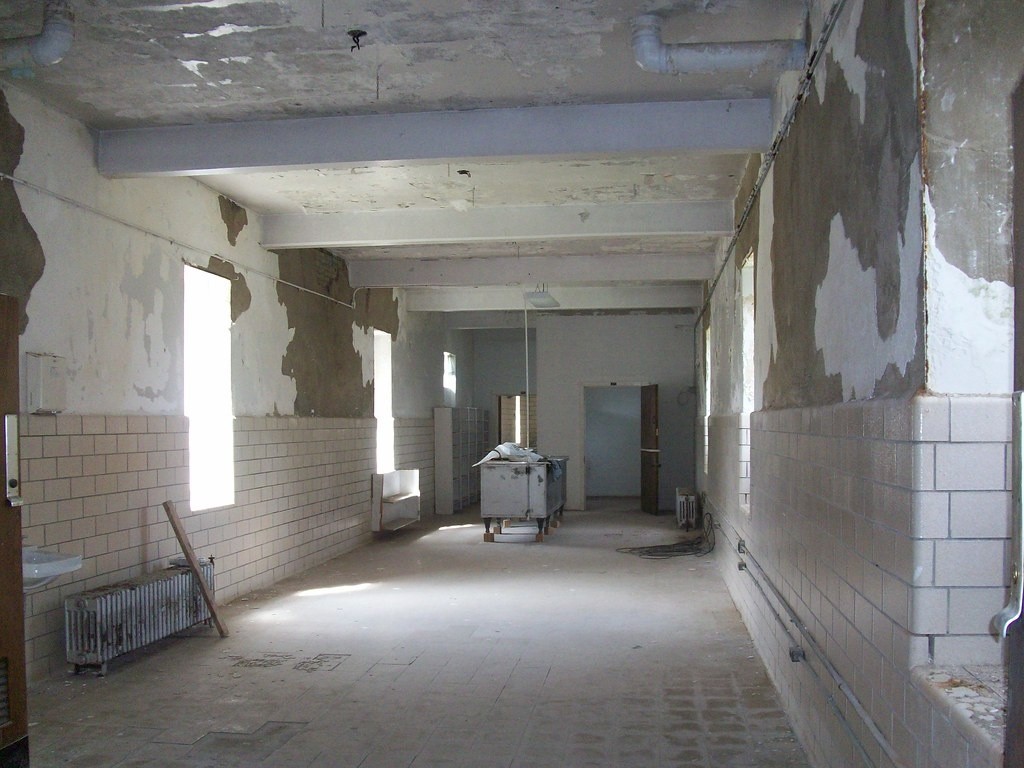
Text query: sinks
22 545 82 593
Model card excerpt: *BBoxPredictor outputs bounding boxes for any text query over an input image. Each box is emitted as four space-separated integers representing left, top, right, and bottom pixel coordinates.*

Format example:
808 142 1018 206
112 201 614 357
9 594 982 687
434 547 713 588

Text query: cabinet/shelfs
371 468 421 532
434 405 490 515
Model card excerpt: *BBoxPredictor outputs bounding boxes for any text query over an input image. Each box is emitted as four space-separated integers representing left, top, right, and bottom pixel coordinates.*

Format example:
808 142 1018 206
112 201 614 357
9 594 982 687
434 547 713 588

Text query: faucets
22 533 33 542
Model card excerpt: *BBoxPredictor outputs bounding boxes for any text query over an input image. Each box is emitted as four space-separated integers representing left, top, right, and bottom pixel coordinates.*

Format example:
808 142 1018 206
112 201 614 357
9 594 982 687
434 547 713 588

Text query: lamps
527 283 560 310
